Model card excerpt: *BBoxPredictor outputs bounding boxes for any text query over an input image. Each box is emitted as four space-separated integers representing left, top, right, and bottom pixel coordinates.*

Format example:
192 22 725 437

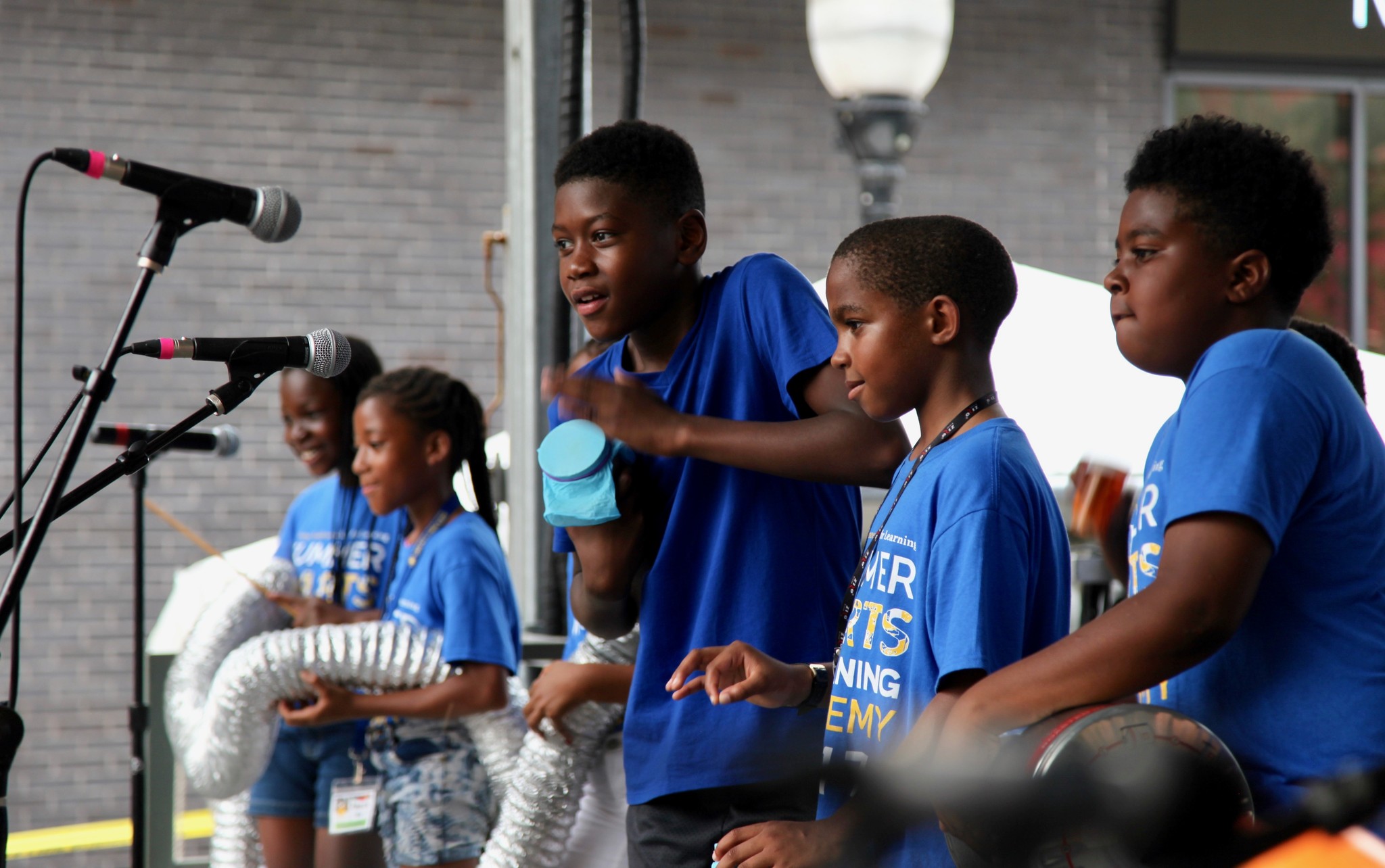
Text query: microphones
49 147 301 244
90 423 240 457
132 328 352 377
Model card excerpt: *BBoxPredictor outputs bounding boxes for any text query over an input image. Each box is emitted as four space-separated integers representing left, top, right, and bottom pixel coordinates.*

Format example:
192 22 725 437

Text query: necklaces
331 475 368 606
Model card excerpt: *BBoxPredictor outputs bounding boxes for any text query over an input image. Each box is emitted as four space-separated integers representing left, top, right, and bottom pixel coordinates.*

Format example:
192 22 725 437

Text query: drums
941 697 1254 868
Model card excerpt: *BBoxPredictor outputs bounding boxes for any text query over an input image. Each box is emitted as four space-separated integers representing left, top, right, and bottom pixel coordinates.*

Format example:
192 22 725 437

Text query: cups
1071 459 1127 544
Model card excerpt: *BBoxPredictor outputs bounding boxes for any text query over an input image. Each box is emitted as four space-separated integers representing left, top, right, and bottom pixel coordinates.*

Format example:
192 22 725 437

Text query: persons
934 114 1385 868
551 118 912 868
247 333 640 868
666 215 1071 868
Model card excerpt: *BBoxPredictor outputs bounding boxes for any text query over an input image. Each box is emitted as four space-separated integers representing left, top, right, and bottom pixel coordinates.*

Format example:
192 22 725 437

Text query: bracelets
797 660 829 716
